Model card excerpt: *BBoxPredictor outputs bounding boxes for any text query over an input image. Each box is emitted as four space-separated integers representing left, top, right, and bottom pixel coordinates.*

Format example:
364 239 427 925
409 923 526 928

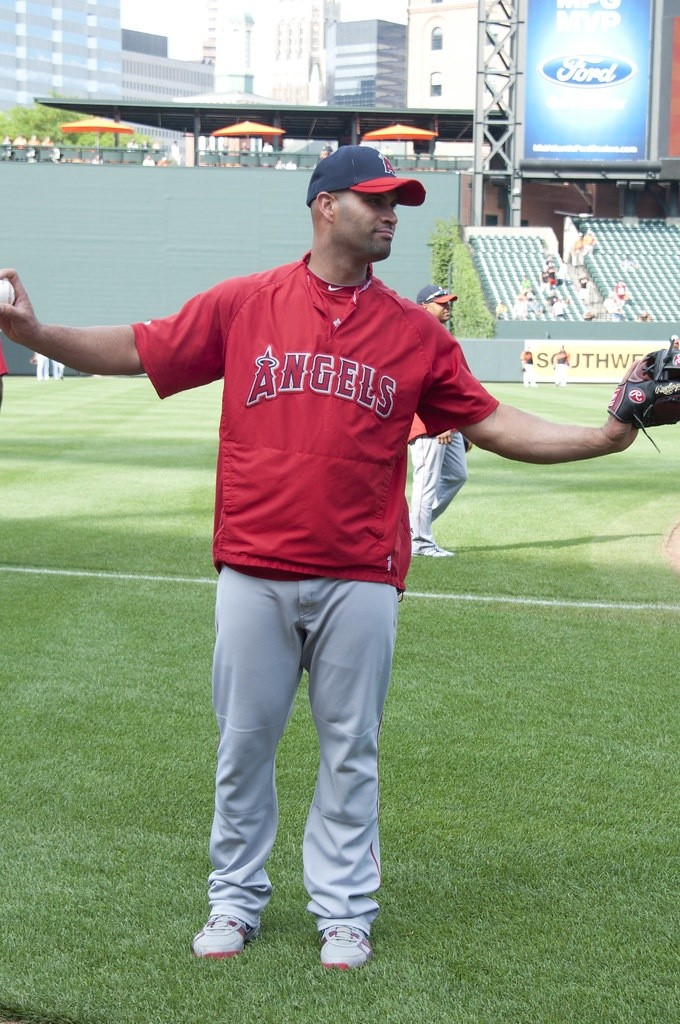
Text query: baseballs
0 275 16 307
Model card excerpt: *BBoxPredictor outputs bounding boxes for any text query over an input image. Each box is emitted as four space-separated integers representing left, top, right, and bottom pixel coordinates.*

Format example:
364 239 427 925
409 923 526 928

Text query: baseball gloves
610 334 680 431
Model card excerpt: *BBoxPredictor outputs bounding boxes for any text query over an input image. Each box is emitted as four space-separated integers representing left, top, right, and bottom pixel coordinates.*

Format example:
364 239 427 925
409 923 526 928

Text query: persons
405 284 474 557
571 230 595 266
620 257 637 271
521 345 533 386
553 345 570 387
0 144 680 969
671 335 679 350
496 254 569 322
263 142 273 152
127 139 161 149
578 275 651 323
142 155 155 166
1 135 55 161
275 159 297 170
31 352 65 380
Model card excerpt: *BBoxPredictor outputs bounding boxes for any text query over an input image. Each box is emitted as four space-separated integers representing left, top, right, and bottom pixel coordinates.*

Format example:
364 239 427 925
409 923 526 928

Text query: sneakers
191 914 260 962
320 925 372 972
411 548 453 557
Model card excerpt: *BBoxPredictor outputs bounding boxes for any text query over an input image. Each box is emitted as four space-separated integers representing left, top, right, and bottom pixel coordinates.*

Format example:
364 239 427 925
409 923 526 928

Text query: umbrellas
211 121 286 151
362 123 438 156
57 115 134 147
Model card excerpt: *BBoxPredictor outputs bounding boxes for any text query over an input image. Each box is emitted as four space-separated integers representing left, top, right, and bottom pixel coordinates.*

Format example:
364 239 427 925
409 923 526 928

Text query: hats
417 286 457 304
307 145 425 208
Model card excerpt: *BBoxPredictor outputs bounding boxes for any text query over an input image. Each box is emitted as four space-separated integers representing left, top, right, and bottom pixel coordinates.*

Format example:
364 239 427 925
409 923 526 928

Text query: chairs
468 216 680 324
